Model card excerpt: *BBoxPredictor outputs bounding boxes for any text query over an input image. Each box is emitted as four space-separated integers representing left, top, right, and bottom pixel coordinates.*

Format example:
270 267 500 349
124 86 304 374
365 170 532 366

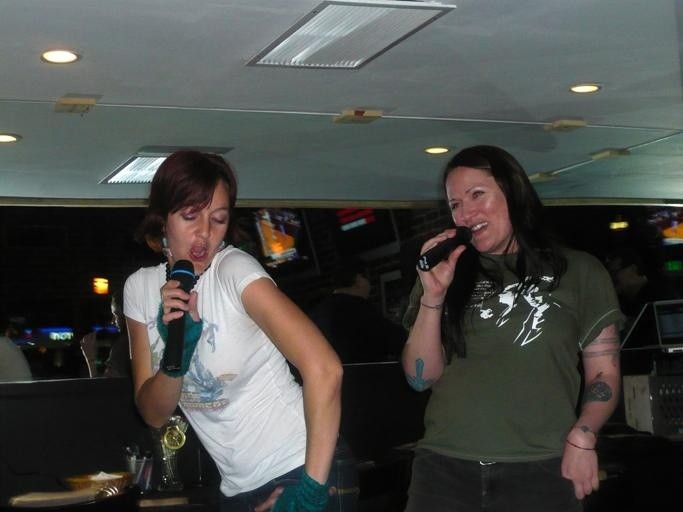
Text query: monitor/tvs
653 299 683 347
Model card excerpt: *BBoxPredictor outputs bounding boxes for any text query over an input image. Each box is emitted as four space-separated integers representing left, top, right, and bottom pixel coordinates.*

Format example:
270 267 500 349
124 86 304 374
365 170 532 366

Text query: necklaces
165 263 211 289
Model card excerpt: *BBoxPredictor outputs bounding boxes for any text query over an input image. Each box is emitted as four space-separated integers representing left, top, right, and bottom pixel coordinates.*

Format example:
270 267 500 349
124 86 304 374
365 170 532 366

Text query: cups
150 414 189 489
127 449 153 495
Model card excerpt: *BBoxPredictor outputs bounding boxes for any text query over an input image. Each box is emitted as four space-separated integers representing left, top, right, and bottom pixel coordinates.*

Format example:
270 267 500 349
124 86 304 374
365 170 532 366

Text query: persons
122 149 345 511
79 287 130 378
603 252 654 375
398 145 628 512
307 266 409 363
0 316 34 382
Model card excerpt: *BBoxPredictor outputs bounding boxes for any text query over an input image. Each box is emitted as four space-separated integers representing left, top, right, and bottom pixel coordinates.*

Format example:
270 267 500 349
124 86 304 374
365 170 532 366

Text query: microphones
416 224 472 271
163 259 196 372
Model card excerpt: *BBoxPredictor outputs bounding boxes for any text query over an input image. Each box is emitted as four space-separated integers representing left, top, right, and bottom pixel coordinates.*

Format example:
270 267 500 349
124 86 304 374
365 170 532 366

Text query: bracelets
420 301 444 309
563 438 597 451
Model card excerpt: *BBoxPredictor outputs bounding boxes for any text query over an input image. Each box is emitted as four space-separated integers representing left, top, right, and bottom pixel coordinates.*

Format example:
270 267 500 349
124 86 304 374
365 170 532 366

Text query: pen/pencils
126 442 154 490
